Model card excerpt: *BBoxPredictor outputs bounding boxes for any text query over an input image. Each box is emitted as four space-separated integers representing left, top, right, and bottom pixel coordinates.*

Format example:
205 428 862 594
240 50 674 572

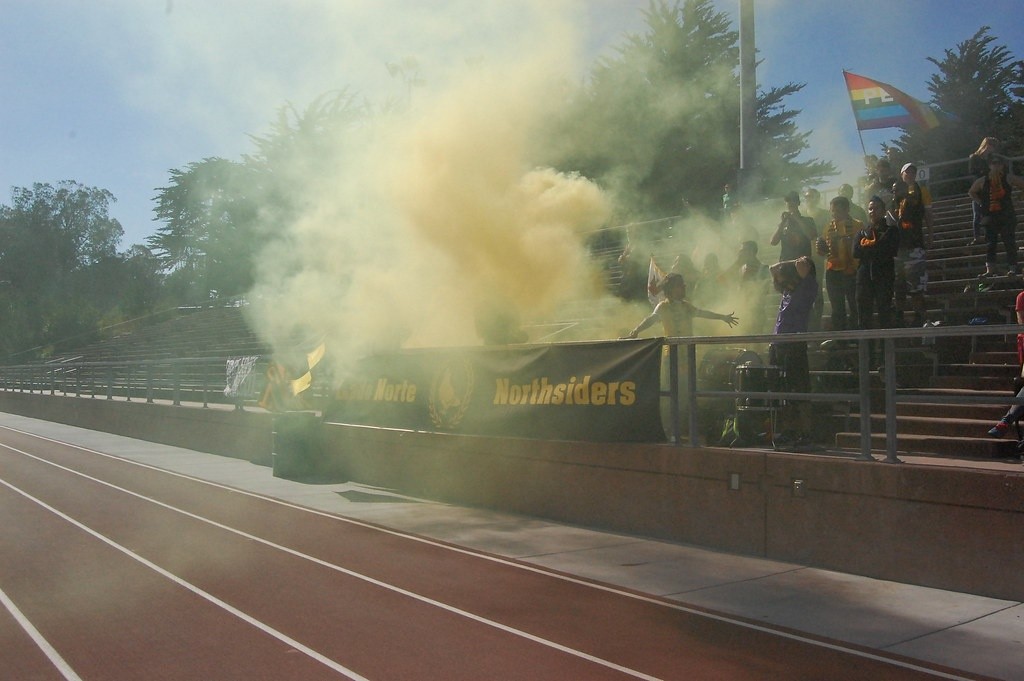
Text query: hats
900 163 916 174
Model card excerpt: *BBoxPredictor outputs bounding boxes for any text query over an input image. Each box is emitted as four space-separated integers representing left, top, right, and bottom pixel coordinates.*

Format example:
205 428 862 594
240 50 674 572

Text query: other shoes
987 417 1010 438
1007 268 1016 276
889 318 905 328
819 339 841 350
978 272 993 278
911 319 927 327
774 432 797 451
847 340 858 348
794 433 814 452
909 247 923 257
967 239 979 246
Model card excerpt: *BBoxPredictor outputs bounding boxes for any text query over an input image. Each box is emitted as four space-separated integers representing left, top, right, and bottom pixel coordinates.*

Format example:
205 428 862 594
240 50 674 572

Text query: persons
771 147 926 376
988 283 1024 438
769 256 817 444
617 179 771 356
968 136 1012 245
631 272 739 445
968 155 1024 277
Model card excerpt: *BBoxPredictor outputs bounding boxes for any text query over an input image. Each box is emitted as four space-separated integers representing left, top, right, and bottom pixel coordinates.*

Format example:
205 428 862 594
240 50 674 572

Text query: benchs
0 171 1024 462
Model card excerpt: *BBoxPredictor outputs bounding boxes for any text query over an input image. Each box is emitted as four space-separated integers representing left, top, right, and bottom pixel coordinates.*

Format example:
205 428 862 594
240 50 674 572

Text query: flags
844 72 940 131
648 258 668 307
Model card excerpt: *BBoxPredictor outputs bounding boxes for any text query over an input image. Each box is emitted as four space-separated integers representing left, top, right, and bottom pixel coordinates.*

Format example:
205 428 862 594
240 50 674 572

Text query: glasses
991 160 1003 166
